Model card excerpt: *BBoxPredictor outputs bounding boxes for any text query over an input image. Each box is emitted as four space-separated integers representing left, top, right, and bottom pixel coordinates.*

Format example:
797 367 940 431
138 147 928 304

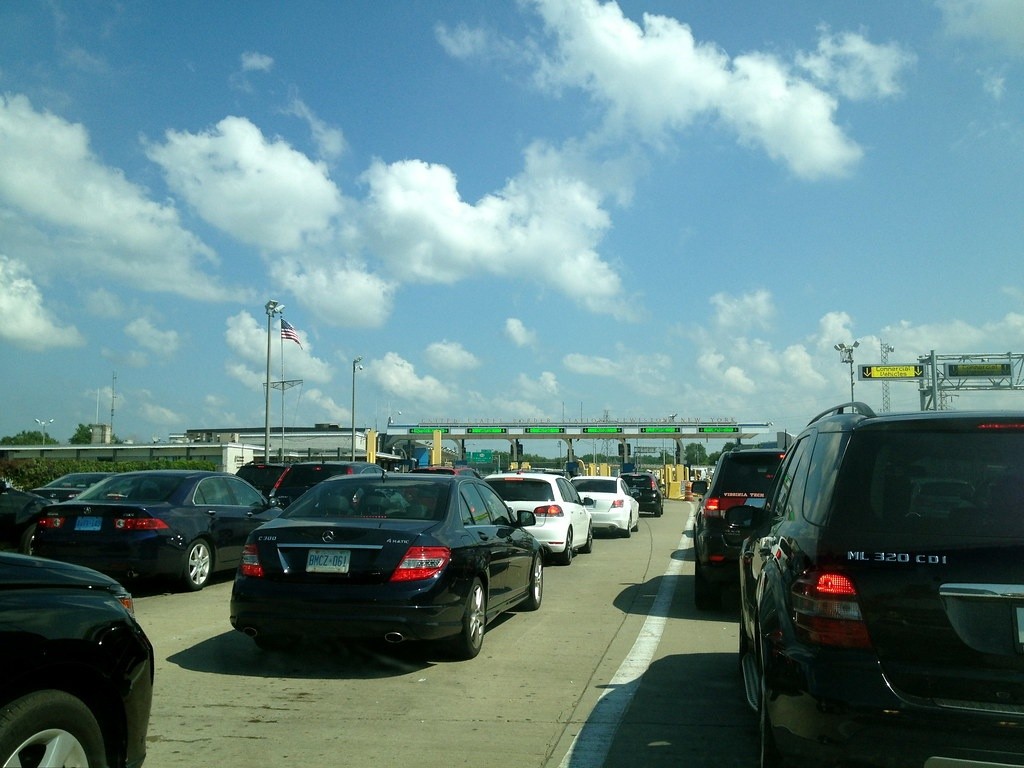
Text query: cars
1 549 155 767
269 460 387 510
0 479 56 556
483 472 595 566
229 473 545 662
32 469 284 593
567 475 640 538
406 466 481 506
619 471 666 518
31 471 119 506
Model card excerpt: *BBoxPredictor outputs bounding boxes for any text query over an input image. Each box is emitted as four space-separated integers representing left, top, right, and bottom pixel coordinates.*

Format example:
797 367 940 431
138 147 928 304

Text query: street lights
262 299 286 465
35 418 54 447
351 355 365 463
834 340 860 414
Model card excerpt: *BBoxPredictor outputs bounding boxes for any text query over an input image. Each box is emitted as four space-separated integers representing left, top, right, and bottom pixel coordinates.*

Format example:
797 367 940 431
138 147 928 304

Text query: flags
281 319 303 351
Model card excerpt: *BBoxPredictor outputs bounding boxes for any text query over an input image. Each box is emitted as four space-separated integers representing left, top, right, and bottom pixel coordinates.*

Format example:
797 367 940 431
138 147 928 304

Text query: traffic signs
857 363 927 380
943 362 1014 378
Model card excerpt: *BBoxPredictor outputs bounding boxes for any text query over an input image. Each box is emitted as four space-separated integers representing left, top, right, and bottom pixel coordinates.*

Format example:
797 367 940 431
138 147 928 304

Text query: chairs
363 492 391 515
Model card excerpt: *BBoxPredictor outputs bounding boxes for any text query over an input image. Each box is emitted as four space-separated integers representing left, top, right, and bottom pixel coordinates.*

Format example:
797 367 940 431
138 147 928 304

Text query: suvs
740 401 1024 767
691 446 790 612
235 459 301 496
